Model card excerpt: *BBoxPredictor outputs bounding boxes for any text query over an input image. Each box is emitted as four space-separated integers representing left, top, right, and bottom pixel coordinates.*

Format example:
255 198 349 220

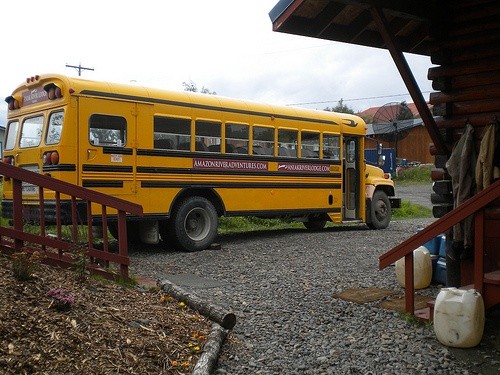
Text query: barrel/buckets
432 288 484 347
395 247 432 291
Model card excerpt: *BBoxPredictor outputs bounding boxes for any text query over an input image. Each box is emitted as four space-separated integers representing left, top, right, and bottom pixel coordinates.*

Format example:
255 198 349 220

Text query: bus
1 75 402 252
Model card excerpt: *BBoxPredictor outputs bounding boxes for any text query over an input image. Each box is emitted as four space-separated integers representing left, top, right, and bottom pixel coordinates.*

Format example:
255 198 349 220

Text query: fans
371 101 415 142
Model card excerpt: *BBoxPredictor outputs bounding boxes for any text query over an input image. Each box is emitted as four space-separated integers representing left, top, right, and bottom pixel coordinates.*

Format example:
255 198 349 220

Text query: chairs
154 139 334 158
